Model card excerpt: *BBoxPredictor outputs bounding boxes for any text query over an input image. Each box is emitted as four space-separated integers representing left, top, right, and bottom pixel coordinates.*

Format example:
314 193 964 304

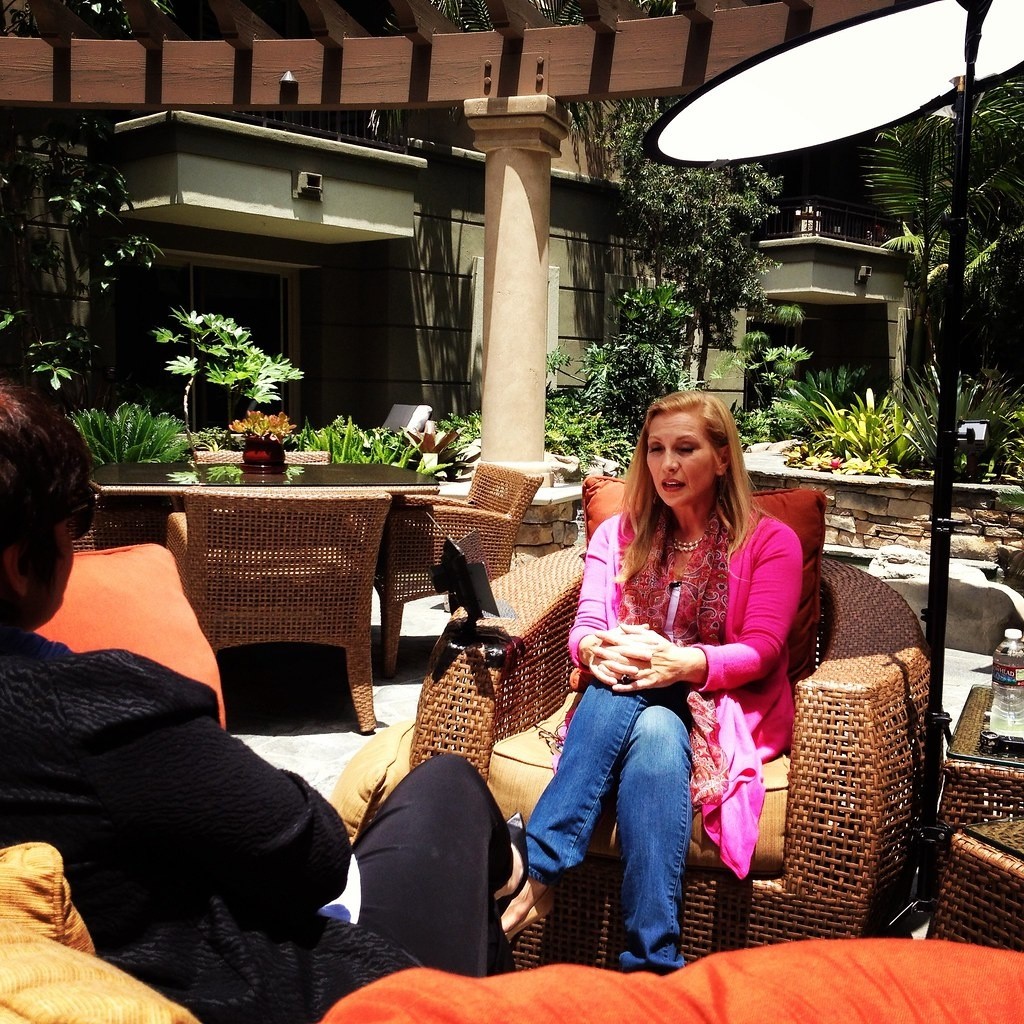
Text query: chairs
380 403 434 433
373 461 546 680
164 486 392 732
407 551 933 975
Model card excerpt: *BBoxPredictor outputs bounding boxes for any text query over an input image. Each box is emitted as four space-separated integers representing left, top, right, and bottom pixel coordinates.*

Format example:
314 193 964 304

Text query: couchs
42 542 226 736
1 936 1024 1024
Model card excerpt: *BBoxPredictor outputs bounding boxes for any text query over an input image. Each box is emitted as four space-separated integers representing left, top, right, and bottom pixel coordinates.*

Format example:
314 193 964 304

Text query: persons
502 392 802 977
0 379 528 1024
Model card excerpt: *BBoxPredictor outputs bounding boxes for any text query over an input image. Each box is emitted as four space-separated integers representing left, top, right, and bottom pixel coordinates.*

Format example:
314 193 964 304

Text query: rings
620 674 630 684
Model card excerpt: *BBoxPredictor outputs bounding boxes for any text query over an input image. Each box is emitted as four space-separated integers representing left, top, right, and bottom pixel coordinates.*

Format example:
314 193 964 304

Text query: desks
931 684 1023 892
90 460 439 546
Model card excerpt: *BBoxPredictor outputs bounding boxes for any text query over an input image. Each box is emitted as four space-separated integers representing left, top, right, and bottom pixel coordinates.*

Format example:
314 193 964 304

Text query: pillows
583 475 826 705
0 841 97 959
327 722 415 849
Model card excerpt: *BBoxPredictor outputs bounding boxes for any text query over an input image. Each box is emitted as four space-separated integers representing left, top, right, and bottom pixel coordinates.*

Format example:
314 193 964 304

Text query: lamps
290 171 323 202
853 264 872 285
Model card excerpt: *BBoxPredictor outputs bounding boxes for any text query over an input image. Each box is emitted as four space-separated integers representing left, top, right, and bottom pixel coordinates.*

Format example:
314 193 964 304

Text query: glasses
51 482 94 538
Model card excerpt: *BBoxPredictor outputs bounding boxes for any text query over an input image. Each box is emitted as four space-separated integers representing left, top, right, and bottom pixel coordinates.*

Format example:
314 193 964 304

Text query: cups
424 420 436 435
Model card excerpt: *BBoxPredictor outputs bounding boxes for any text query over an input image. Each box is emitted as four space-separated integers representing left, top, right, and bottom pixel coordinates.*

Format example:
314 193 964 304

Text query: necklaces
673 537 704 551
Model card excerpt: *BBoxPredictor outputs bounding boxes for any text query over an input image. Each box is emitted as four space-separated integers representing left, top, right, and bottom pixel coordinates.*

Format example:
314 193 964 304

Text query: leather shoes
494 812 528 915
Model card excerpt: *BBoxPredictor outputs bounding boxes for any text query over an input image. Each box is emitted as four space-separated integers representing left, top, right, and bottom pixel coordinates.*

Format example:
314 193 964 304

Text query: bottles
990 628 1024 738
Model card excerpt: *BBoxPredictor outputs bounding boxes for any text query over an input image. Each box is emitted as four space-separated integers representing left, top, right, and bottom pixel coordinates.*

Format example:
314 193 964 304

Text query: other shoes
504 888 555 941
